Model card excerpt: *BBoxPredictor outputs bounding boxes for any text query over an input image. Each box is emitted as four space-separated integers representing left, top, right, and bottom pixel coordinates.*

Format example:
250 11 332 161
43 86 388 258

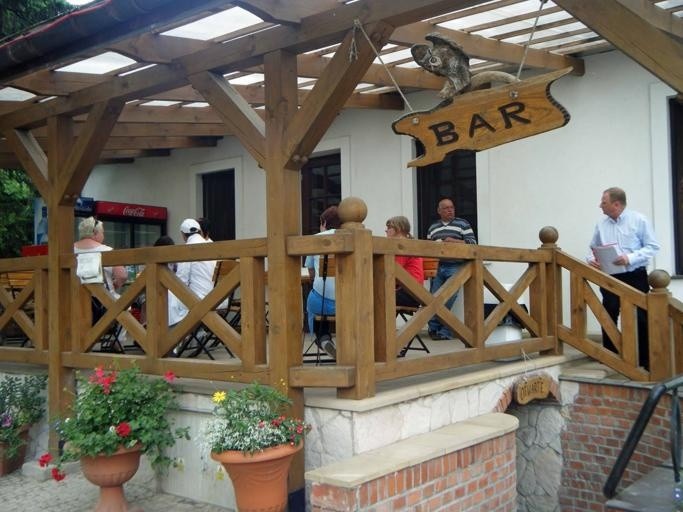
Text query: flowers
206 374 313 458
37 359 191 480
0 372 47 462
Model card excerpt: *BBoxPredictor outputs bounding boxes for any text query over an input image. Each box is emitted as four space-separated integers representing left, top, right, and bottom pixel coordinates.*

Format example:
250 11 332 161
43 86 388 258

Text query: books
589 242 628 277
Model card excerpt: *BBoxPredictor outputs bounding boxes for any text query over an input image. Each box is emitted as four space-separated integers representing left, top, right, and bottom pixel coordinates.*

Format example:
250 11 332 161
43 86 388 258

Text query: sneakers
320 341 337 359
427 322 447 340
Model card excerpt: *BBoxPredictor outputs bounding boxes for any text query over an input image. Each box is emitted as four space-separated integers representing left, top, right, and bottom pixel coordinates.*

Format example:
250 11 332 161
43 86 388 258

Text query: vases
0 422 33 478
80 442 144 512
210 433 309 512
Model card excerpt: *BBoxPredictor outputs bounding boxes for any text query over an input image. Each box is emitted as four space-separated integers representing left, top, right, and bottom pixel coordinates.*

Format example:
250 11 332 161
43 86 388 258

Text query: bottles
36 206 49 246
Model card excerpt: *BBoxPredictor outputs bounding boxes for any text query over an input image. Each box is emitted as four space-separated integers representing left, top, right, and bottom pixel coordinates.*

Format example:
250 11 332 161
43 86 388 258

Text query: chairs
0 253 441 366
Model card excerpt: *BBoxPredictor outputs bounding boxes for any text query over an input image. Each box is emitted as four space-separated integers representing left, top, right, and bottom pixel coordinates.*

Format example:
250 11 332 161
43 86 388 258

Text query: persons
74 215 230 355
426 198 476 341
585 187 661 372
384 215 425 308
304 206 341 359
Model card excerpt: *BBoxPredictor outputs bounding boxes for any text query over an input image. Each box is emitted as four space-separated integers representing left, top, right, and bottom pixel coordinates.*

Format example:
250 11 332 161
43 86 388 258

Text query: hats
181 219 201 234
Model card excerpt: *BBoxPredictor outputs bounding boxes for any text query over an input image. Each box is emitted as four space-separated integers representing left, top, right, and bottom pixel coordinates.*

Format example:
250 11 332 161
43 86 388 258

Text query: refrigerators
33 196 169 324
